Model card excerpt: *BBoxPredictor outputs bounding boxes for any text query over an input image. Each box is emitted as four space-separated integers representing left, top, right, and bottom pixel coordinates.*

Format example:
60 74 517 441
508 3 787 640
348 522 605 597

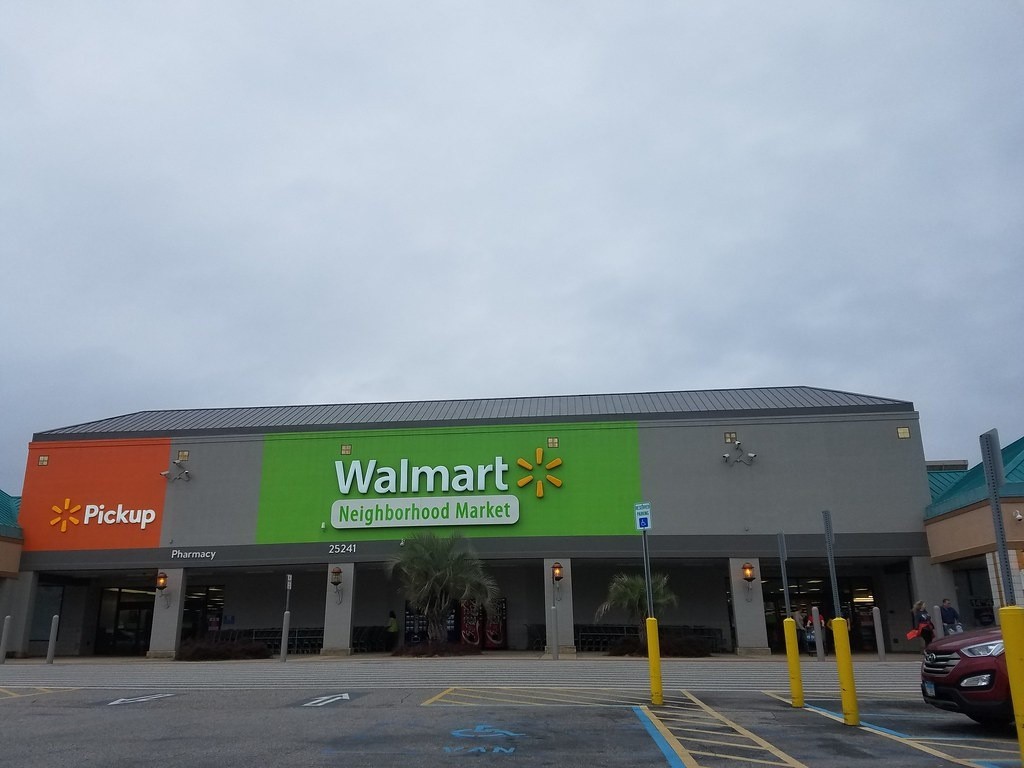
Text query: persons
828 606 851 631
807 612 824 627
383 610 399 652
913 600 935 645
793 607 805 641
940 598 959 636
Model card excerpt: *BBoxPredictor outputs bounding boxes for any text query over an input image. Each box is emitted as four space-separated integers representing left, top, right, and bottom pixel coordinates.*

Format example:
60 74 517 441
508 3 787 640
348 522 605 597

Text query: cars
920 625 1016 727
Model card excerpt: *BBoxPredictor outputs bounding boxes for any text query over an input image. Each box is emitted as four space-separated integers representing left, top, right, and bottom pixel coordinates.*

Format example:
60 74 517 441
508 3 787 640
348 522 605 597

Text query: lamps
741 562 756 590
153 572 169 597
551 562 565 588
330 567 343 594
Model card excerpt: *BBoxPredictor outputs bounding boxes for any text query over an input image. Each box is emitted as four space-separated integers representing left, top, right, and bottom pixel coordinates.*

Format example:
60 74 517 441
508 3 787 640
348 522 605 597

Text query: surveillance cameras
185 471 189 475
160 471 170 476
735 441 741 445
748 453 757 457
723 454 729 459
1016 514 1023 522
173 460 181 465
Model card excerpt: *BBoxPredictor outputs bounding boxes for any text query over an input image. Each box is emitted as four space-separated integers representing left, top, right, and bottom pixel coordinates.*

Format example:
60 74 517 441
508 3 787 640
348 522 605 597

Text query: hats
802 608 808 613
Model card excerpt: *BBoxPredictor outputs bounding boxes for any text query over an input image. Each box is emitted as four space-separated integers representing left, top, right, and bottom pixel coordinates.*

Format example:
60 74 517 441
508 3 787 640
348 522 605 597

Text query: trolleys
803 626 829 657
945 624 966 634
